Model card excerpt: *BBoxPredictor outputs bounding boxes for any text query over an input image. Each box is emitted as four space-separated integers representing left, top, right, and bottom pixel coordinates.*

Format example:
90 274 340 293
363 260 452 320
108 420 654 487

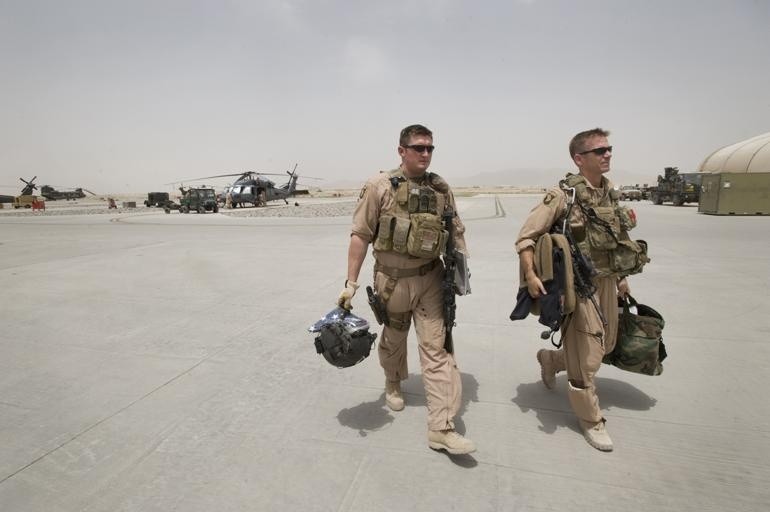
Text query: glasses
578 145 613 155
400 144 434 153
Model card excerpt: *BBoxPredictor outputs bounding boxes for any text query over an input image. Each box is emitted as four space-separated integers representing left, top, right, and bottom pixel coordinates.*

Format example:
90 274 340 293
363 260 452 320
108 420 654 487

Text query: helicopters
0 176 38 204
41 186 85 201
162 164 323 207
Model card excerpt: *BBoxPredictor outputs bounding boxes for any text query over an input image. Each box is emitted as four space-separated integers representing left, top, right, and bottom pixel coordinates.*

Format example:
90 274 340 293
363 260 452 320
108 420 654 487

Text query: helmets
308 308 378 369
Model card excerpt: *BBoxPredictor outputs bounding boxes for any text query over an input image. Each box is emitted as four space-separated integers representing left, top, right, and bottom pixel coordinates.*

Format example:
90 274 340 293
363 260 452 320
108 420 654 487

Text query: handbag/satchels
518 233 576 316
379 212 449 257
599 292 667 375
585 207 649 275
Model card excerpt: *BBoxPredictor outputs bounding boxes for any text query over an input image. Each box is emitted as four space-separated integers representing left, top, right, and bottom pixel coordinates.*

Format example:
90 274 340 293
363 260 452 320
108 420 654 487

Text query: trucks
618 185 642 201
144 188 218 214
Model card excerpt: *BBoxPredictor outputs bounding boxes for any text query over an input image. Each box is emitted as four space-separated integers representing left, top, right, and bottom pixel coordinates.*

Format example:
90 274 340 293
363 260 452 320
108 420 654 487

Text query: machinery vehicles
14 194 39 208
650 167 699 206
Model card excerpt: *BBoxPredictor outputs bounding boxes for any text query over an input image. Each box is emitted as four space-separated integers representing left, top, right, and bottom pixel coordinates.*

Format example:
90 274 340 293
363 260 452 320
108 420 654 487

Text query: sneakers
578 414 614 452
427 427 477 456
538 349 557 390
384 378 405 411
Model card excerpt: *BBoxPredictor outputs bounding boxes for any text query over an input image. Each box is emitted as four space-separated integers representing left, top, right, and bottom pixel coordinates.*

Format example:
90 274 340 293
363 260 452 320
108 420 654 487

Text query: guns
442 211 457 355
550 224 609 325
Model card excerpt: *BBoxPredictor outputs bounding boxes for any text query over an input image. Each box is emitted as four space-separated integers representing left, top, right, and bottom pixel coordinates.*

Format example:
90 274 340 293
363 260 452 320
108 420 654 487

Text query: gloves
336 281 360 311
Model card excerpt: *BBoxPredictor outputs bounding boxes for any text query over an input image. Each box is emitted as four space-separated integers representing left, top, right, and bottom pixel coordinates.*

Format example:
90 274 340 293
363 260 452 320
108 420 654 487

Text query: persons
338 126 476 456
514 128 631 451
258 193 264 207
225 192 233 209
